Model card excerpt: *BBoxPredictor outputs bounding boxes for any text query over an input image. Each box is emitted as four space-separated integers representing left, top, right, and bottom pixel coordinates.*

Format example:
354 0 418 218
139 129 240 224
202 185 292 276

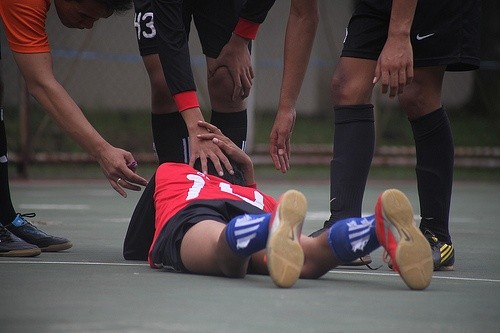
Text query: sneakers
375 189 433 289
308 220 383 270
0 224 42 257
266 189 307 288
4 212 73 251
389 228 454 270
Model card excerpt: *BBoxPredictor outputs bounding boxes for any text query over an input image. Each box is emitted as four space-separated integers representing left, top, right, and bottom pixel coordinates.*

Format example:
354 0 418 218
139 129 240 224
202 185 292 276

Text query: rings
116 179 121 182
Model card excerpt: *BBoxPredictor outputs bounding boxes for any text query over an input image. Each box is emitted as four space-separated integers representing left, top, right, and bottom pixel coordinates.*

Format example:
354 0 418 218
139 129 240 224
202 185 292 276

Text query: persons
123 120 434 289
270 0 483 270
134 0 276 178
0 0 149 258
